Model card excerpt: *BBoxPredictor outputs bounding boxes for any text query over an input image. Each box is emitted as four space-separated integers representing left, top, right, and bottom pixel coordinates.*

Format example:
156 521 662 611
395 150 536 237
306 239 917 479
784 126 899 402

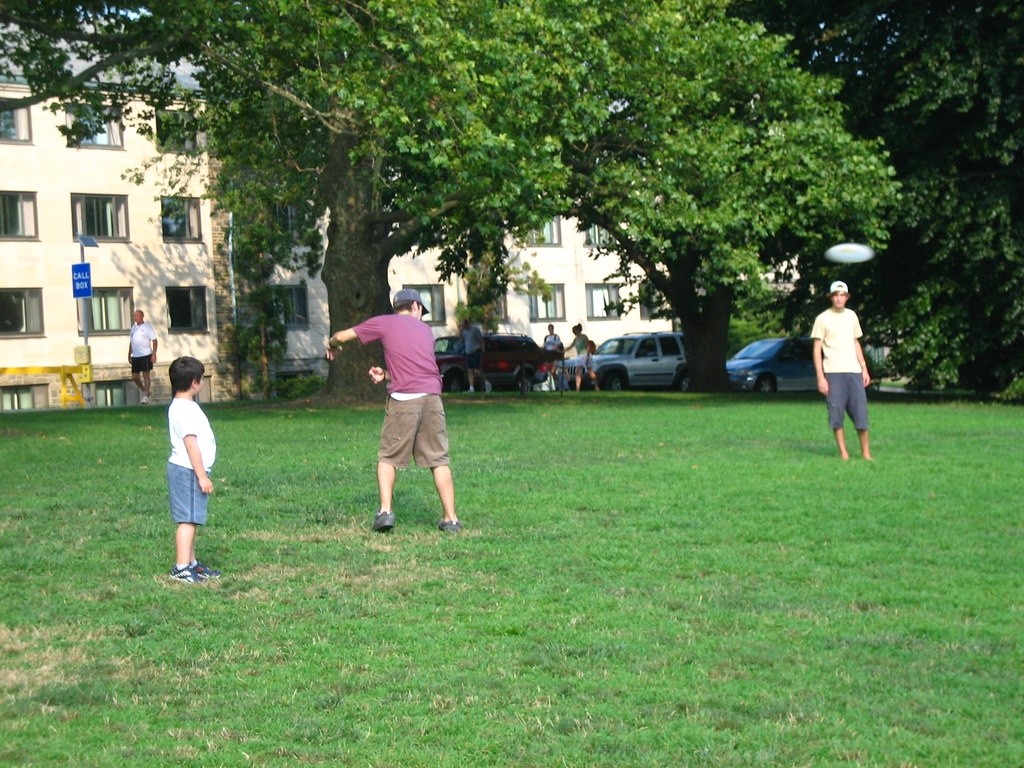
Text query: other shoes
468 389 474 393
142 397 149 404
485 383 492 392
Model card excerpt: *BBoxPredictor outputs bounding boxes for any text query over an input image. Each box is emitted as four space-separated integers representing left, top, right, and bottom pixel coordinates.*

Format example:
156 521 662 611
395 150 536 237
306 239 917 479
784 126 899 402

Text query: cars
726 334 819 396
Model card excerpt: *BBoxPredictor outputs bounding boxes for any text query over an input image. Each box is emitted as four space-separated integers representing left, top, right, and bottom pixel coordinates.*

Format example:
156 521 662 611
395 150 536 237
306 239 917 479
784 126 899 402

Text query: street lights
78 233 102 405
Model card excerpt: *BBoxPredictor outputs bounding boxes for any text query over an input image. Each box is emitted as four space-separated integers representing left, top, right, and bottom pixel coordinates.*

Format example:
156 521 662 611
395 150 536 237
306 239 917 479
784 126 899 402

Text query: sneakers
169 564 205 584
193 560 221 579
438 519 461 531
373 511 394 531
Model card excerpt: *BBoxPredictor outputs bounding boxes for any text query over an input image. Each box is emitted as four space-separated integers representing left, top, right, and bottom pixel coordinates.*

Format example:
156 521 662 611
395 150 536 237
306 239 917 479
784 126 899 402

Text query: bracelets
328 342 337 348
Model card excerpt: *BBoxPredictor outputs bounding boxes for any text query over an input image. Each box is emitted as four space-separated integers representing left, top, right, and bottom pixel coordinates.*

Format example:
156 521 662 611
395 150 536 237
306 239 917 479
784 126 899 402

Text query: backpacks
544 334 563 351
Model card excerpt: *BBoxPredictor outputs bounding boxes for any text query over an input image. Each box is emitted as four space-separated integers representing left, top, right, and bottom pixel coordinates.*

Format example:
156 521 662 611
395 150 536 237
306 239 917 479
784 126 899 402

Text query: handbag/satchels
589 341 595 353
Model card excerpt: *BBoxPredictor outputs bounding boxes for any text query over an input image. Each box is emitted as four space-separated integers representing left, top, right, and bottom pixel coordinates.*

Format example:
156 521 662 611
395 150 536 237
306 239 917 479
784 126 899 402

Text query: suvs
434 332 551 393
551 331 693 392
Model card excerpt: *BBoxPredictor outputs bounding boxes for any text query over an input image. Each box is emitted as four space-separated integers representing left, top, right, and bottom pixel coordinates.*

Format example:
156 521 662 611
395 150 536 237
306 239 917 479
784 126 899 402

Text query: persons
128 310 158 404
326 290 464 533
166 356 222 584
811 280 872 462
542 324 602 392
458 318 493 393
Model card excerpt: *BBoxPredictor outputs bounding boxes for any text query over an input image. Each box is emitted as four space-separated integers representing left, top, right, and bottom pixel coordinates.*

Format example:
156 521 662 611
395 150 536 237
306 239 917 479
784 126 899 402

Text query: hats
394 289 429 315
830 281 848 293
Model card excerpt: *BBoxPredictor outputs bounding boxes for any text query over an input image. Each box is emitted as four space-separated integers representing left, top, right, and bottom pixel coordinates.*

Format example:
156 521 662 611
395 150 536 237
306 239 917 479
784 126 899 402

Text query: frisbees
824 244 874 265
